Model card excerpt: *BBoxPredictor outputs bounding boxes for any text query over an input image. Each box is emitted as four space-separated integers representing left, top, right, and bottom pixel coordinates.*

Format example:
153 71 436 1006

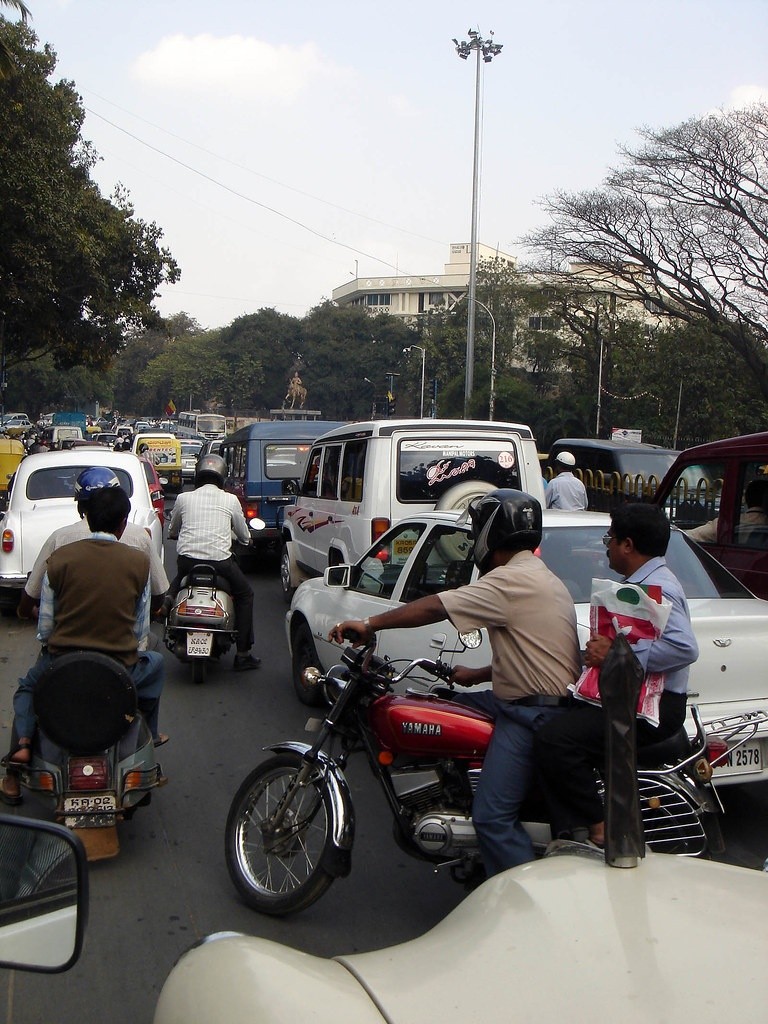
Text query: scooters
8 566 168 863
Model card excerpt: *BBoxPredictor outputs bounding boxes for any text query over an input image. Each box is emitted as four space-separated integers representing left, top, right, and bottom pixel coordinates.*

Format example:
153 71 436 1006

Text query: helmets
555 451 576 470
469 489 543 568
195 454 229 485
138 443 148 453
74 466 121 502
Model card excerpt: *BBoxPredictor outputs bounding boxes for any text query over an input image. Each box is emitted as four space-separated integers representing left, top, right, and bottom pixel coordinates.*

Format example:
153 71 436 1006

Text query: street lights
465 297 496 420
401 344 425 419
451 23 503 420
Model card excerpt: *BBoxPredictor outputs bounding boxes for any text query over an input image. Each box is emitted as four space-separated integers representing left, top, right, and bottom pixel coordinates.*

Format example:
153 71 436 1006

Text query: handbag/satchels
573 578 671 727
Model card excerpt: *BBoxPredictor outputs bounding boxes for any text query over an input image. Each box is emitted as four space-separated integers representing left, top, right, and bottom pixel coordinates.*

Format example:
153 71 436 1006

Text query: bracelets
363 616 371 632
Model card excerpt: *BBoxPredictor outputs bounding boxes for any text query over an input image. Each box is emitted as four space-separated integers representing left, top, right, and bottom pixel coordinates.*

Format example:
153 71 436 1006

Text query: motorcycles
223 626 768 918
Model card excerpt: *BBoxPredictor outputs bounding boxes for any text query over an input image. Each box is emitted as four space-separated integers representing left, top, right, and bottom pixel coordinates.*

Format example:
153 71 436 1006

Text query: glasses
601 532 617 546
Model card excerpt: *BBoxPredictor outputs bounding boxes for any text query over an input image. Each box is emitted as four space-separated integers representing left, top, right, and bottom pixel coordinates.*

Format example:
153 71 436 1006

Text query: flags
163 400 177 416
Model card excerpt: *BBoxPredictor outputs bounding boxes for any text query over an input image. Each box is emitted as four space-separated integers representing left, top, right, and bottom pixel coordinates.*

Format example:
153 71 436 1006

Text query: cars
0 402 246 617
285 507 768 793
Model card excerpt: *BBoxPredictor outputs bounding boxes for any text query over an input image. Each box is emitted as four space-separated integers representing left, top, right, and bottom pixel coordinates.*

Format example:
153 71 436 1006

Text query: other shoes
234 654 261 670
157 605 169 618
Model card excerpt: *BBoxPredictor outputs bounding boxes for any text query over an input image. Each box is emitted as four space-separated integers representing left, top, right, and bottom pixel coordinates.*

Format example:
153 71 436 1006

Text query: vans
651 431 768 601
547 438 722 522
279 417 547 610
218 420 350 574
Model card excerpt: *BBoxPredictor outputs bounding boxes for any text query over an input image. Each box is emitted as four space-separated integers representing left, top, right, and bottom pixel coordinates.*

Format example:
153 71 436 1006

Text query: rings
337 623 340 627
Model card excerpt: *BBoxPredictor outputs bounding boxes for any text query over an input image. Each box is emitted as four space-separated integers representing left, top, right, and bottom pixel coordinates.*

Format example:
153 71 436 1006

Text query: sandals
1 743 30 768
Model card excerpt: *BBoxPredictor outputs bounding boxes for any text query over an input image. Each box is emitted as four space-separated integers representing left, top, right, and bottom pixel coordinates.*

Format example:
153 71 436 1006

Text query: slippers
154 732 169 747
0 776 24 806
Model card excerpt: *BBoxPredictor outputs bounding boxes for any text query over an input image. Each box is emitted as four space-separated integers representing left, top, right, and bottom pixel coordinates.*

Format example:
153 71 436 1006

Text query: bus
176 411 228 444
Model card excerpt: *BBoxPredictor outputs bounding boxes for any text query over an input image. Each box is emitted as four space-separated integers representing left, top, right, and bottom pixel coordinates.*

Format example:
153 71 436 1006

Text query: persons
1 468 170 808
114 431 159 465
544 451 588 511
0 424 51 453
328 489 698 884
291 372 302 396
164 454 264 672
133 417 163 429
683 480 768 545
87 415 117 433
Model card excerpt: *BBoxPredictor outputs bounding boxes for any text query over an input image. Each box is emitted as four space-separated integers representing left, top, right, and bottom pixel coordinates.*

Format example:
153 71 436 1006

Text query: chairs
564 579 583 601
747 528 768 550
295 454 306 465
